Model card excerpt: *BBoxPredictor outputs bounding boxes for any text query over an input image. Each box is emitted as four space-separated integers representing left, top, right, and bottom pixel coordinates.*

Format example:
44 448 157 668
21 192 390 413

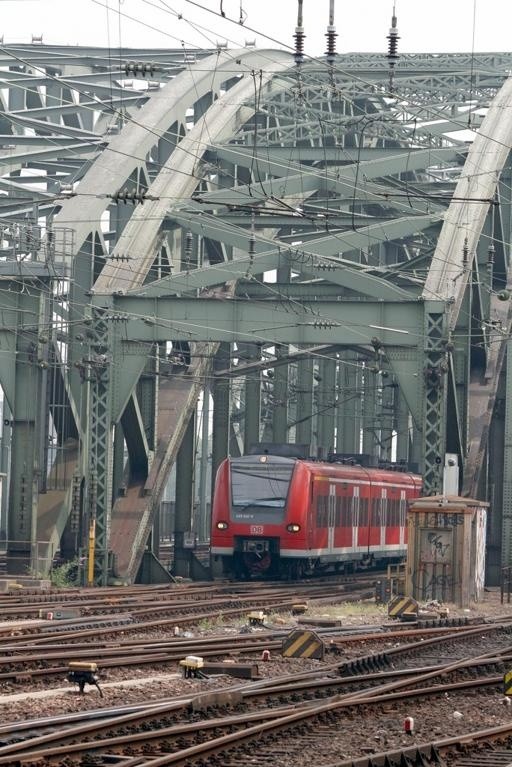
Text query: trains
195 455 425 573
